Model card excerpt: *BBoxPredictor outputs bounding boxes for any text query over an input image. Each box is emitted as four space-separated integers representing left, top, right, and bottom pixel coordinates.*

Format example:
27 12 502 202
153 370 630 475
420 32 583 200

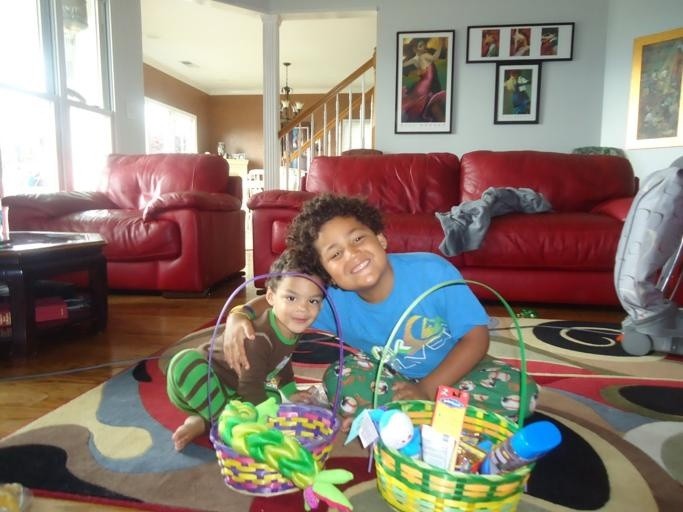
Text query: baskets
207 273 343 497
372 279 537 511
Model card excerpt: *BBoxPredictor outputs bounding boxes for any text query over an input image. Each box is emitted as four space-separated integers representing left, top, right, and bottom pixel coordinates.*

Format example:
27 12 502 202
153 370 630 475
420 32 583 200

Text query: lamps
280 63 305 124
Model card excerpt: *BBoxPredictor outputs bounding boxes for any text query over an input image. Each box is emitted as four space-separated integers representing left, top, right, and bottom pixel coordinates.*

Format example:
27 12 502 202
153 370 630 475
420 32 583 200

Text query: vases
217 142 225 157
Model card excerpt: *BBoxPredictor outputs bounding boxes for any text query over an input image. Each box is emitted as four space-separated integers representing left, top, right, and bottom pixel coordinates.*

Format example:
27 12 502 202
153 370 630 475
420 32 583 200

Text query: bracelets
229 304 258 323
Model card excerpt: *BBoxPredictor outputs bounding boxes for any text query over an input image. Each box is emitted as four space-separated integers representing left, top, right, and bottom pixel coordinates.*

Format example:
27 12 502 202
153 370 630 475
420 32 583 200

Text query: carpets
0 311 683 512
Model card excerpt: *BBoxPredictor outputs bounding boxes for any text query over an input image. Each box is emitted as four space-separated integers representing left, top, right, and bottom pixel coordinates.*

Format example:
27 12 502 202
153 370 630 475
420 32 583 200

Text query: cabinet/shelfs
222 158 248 197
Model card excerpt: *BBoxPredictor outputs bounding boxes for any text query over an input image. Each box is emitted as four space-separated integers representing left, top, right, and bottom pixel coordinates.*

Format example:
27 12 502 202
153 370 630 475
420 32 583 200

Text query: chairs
0 151 246 300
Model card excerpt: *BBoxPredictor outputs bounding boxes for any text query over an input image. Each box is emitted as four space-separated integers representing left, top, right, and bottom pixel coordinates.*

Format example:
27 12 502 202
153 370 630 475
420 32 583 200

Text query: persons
223 190 539 432
507 70 530 113
403 38 446 122
167 249 328 455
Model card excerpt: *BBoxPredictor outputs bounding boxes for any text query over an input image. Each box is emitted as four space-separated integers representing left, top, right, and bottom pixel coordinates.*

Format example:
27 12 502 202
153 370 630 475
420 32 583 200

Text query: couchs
245 151 683 307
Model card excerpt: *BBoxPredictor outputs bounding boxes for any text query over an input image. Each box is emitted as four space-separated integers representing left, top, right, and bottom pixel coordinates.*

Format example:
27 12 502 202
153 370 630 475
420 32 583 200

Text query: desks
0 231 111 359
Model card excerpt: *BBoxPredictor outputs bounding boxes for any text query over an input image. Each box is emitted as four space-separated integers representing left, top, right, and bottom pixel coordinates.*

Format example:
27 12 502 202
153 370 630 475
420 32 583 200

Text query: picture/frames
625 27 683 150
395 30 455 134
466 22 575 63
494 60 542 124
282 126 310 174
